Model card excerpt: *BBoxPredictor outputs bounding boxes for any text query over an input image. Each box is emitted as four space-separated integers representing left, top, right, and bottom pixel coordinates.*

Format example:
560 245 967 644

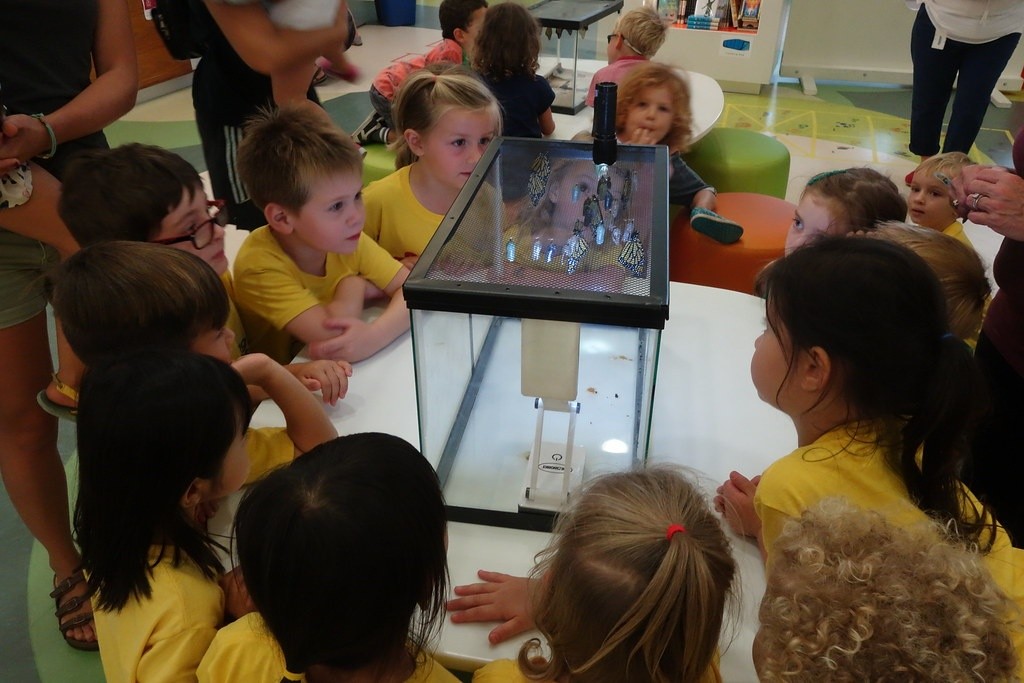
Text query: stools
672 193 800 301
691 128 792 203
359 142 402 191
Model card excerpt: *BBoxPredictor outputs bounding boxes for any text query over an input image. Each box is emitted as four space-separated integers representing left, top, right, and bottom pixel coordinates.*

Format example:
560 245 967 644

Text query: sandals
38 373 79 422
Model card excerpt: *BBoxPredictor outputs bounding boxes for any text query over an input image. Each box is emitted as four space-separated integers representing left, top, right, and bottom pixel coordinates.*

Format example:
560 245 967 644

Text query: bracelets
33 110 58 160
972 193 984 211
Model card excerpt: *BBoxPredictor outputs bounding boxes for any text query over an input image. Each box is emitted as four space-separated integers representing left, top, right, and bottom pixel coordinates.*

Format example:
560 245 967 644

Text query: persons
752 499 1024 683
499 150 647 293
615 61 694 156
785 118 1024 548
0 0 556 651
586 5 744 244
904 0 1024 187
713 236 1024 683
193 432 464 683
443 460 742 683
71 353 340 683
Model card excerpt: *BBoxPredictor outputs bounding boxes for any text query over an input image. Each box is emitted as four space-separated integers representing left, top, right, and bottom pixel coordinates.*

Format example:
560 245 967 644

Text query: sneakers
350 109 387 145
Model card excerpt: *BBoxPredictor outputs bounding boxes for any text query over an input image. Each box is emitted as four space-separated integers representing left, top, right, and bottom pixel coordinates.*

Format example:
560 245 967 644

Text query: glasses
151 200 232 249
606 33 625 45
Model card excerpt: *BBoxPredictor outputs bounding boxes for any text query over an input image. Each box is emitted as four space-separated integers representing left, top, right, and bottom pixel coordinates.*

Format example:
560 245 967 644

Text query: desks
527 56 724 153
206 264 798 683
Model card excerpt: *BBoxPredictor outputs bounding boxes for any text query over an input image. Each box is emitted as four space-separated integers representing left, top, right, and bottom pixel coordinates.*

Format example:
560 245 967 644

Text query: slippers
49 567 100 652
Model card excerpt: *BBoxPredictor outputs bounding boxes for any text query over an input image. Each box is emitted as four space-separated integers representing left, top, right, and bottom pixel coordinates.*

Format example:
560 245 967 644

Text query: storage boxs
525 0 625 116
401 138 671 533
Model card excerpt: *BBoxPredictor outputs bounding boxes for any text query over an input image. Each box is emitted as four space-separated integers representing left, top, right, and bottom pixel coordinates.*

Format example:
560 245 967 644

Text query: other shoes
690 207 744 245
904 171 915 187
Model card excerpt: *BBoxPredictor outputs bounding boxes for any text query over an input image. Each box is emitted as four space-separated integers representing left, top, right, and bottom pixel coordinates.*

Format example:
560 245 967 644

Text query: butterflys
617 230 644 277
572 170 632 235
528 151 550 207
561 222 589 276
